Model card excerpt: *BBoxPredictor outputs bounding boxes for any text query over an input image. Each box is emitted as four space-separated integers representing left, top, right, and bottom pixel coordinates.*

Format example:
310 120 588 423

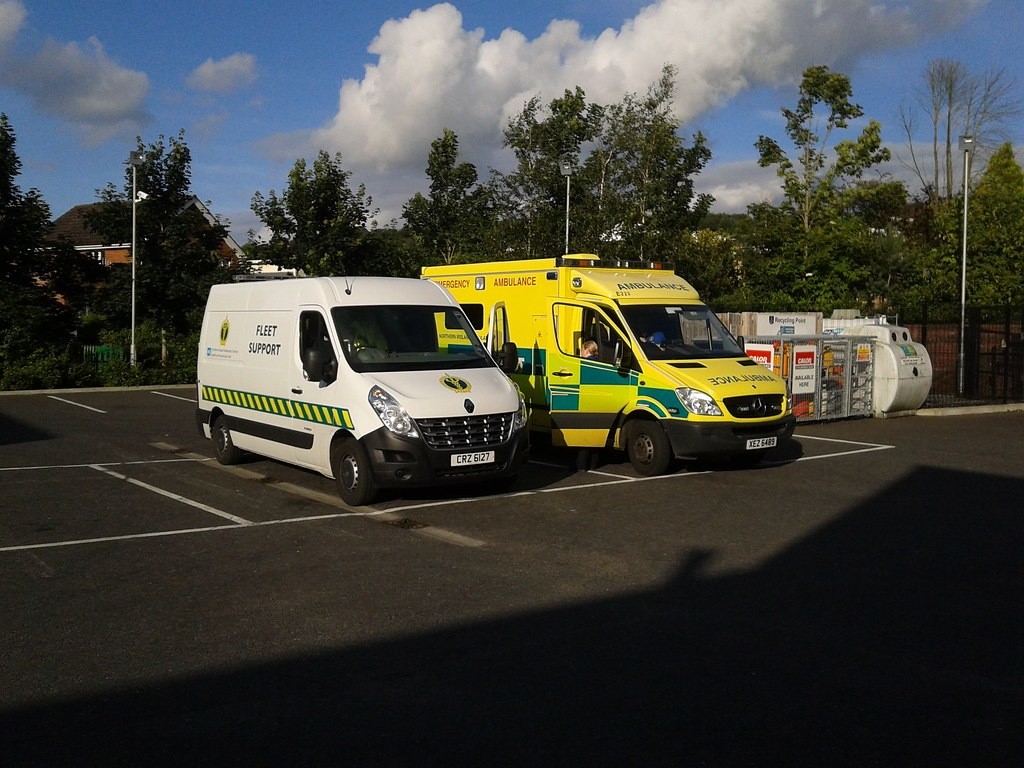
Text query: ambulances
420 253 797 477
195 272 529 507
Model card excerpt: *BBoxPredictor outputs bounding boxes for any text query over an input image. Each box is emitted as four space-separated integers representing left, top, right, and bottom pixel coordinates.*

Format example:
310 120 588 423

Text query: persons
639 328 666 351
573 339 602 472
351 312 389 351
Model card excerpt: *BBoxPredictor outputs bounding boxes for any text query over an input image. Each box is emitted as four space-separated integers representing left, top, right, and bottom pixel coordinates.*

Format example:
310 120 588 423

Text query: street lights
558 160 572 254
957 133 978 396
121 148 147 375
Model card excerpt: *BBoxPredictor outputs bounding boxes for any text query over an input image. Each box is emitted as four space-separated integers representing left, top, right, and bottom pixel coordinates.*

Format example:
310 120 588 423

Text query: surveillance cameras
137 191 150 200
